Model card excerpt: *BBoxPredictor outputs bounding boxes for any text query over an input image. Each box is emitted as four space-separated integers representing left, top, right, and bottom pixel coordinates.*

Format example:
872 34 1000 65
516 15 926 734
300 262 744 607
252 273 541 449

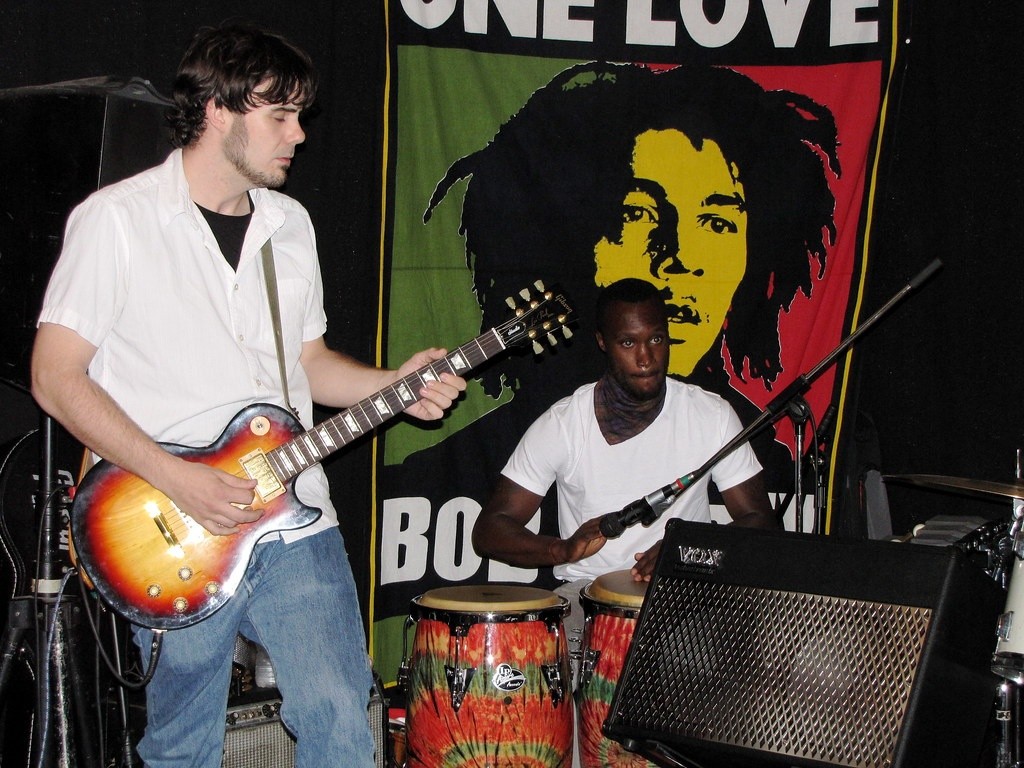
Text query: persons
30 11 466 768
472 277 778 768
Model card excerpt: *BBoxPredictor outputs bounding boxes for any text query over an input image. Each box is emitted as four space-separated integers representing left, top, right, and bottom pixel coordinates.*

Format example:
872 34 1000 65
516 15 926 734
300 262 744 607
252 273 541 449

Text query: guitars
68 277 576 635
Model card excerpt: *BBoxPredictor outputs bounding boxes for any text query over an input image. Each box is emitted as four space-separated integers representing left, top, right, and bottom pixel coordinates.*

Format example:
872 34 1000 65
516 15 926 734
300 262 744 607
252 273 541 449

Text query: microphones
600 473 694 538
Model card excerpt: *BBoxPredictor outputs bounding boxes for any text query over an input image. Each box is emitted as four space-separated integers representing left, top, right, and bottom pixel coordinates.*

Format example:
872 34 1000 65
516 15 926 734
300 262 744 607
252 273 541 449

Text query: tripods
0 410 100 768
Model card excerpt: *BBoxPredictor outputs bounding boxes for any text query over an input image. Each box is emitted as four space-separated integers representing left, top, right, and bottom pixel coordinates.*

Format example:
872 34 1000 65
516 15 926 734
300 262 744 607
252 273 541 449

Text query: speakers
218 686 386 768
600 518 1005 768
0 76 188 391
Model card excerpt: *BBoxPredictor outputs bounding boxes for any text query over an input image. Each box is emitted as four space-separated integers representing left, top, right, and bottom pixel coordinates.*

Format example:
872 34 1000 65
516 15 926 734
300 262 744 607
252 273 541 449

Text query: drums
404 584 573 768
576 570 669 768
